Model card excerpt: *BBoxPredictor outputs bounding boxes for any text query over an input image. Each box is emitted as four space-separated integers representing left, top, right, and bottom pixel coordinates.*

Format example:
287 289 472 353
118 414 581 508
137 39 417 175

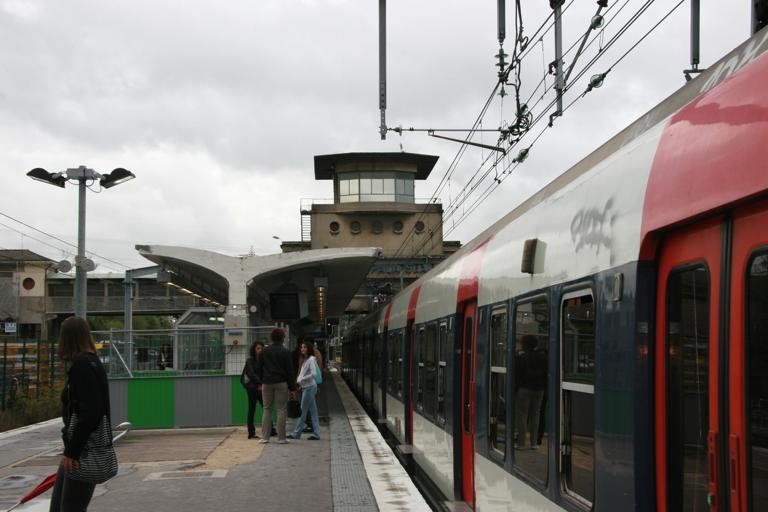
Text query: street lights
62 415 118 486
240 363 259 391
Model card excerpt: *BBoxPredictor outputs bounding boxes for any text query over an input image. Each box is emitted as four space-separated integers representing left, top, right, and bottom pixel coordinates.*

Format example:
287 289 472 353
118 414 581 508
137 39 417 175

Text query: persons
157 344 173 370
50 316 118 512
257 329 323 443
514 335 548 450
240 341 277 439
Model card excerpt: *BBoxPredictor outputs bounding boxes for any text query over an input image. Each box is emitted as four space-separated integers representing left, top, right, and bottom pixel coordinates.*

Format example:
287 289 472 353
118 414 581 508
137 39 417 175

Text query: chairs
248 430 319 443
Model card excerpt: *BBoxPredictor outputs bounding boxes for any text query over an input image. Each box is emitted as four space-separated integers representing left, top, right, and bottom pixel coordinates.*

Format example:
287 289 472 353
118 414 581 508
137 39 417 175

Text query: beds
6 422 131 512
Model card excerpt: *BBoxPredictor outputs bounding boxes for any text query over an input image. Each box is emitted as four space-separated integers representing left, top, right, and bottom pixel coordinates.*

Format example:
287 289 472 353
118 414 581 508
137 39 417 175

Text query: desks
25 165 136 316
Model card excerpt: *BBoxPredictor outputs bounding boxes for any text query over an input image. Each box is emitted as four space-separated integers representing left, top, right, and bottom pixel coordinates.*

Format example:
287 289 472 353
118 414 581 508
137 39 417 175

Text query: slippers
307 358 322 384
